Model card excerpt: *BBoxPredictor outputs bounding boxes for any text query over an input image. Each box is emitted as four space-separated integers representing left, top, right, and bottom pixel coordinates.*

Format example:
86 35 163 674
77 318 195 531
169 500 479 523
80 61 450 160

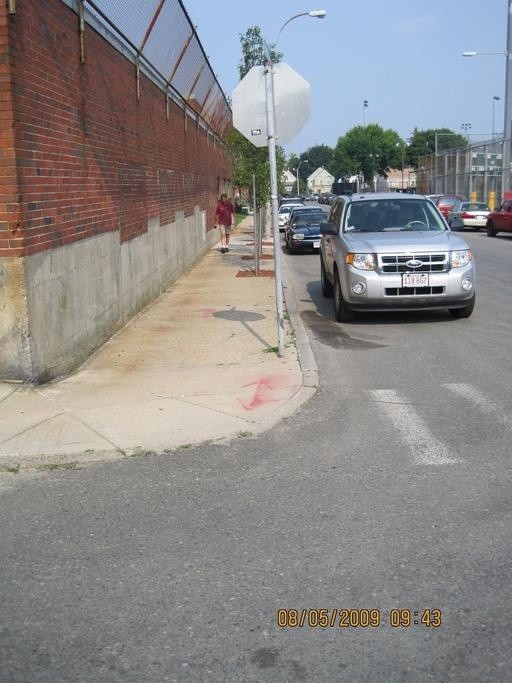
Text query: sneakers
220 247 229 253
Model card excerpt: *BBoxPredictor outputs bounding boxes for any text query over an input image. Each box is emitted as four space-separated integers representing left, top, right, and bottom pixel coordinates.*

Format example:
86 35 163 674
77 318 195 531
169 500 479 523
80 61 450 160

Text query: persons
213 192 235 253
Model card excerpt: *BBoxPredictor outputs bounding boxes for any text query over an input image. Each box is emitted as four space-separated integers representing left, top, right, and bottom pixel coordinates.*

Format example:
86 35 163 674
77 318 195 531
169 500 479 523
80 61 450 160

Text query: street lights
370 154 380 192
395 142 411 191
296 160 308 195
460 0 512 199
262 5 326 360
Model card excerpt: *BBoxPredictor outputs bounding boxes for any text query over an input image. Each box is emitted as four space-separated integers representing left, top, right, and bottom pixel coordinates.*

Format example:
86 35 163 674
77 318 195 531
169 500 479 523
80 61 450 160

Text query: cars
320 192 476 323
425 194 512 238
278 191 340 255
